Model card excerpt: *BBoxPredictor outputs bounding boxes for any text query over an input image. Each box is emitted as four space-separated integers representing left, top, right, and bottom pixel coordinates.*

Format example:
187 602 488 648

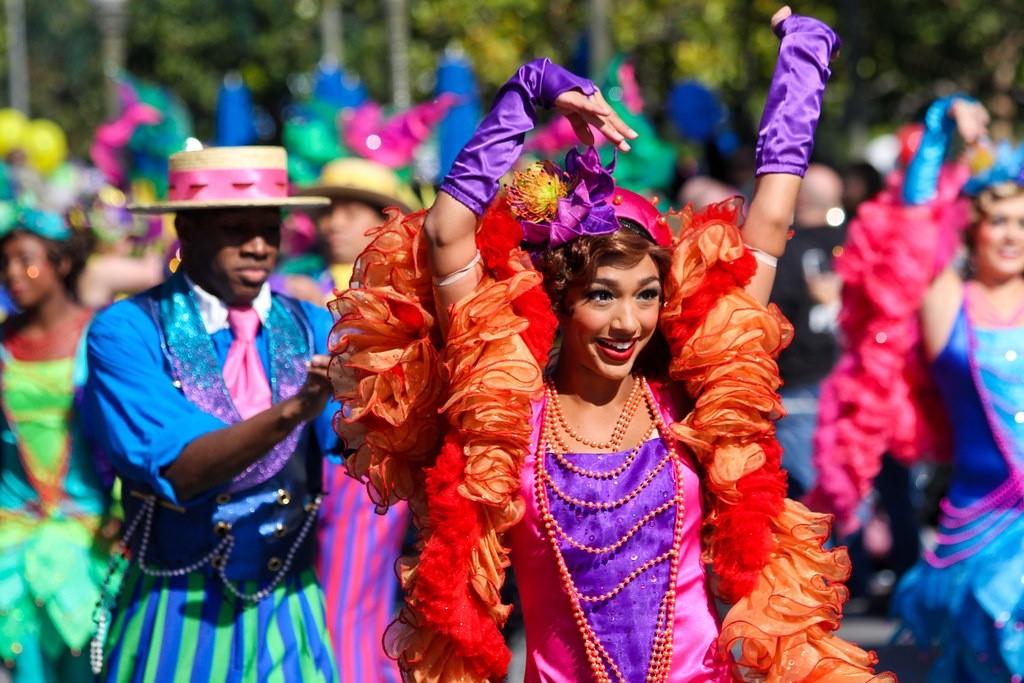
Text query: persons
425 6 840 683
770 91 1024 683
0 145 426 683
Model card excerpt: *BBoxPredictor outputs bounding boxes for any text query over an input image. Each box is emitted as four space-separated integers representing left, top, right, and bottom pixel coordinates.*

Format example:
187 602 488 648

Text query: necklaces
537 368 684 683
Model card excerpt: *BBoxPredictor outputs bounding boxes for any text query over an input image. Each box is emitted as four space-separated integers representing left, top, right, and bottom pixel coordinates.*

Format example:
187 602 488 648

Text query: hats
126 147 332 211
296 156 422 214
504 159 676 258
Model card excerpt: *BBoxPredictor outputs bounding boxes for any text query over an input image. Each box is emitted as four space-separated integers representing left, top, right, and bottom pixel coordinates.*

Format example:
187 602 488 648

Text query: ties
221 307 273 421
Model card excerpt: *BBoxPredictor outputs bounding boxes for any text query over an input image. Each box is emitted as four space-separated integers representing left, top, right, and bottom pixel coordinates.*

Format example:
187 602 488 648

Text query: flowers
504 145 622 250
